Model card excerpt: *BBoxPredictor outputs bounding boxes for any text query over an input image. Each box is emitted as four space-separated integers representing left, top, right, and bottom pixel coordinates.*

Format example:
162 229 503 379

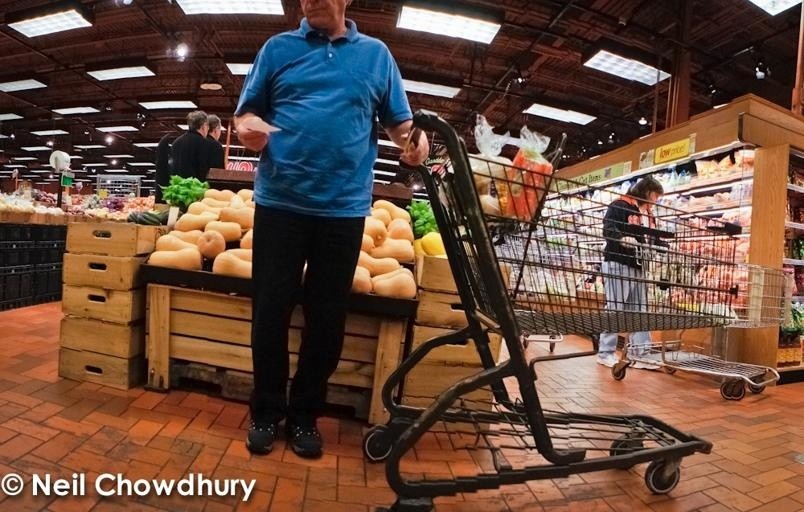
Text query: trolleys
359 105 748 512
601 242 786 402
520 330 567 353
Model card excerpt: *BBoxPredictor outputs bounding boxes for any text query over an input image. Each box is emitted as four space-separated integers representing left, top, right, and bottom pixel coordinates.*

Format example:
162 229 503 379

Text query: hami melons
421 230 447 258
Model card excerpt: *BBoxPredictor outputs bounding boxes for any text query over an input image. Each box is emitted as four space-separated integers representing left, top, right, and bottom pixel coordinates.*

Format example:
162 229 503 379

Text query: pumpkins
146 188 417 299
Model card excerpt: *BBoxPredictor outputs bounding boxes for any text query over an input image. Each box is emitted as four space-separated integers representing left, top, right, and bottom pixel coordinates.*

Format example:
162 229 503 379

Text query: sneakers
629 361 660 369
245 417 279 453
285 419 324 458
596 354 618 368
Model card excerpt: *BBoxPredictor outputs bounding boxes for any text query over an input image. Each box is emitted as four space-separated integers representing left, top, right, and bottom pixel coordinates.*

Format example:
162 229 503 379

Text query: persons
170 110 216 182
232 0 431 458
202 115 227 168
594 173 664 370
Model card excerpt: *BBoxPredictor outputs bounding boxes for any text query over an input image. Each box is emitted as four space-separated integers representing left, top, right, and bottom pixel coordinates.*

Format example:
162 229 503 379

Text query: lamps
174 0 286 16
84 57 158 83
579 36 672 88
222 57 255 77
0 72 50 93
394 0 505 46
519 94 599 127
398 67 464 100
5 0 93 38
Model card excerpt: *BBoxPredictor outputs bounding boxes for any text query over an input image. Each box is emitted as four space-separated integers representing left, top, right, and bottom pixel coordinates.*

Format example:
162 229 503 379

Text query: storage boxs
399 237 511 434
58 223 168 390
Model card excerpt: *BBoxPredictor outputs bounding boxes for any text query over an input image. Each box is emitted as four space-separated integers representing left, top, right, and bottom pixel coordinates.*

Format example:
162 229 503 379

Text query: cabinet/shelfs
494 145 755 380
773 144 804 372
